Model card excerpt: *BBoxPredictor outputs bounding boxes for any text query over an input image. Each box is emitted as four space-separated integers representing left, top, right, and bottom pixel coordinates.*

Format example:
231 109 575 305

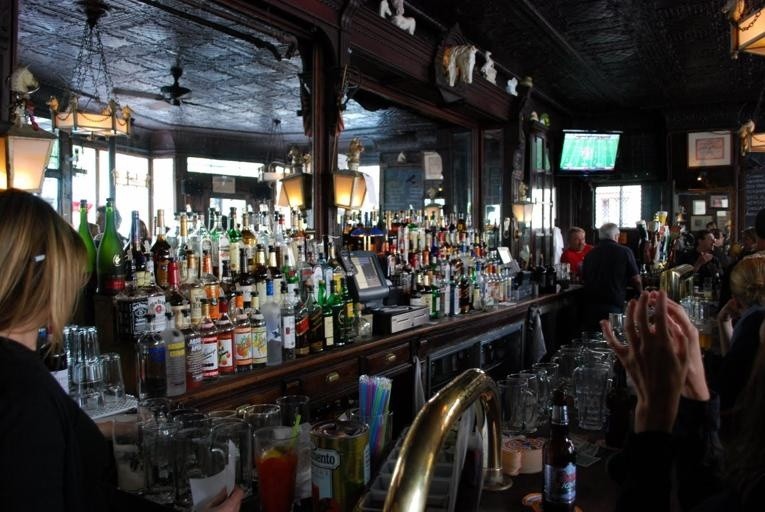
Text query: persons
125 219 149 253
0 187 245 512
561 209 765 512
91 206 121 246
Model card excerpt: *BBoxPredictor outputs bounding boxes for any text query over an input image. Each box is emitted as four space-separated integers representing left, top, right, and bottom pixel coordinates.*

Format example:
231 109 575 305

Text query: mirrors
480 133 505 244
332 68 480 240
0 1 317 251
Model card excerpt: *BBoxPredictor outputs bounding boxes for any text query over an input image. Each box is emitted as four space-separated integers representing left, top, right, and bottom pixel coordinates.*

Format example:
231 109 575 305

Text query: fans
111 58 244 114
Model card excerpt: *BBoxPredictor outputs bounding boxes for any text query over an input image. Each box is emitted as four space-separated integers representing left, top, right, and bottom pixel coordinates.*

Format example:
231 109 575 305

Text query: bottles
649 256 654 276
541 394 577 510
639 263 647 277
78 197 512 395
512 254 556 295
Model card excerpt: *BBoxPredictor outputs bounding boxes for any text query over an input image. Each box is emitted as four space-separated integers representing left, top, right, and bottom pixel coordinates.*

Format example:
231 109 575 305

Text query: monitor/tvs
340 251 390 303
553 127 624 174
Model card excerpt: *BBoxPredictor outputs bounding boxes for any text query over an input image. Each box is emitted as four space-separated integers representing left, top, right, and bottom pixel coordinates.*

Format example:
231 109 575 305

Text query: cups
680 282 714 324
555 262 570 279
62 327 127 410
495 312 629 434
114 399 394 511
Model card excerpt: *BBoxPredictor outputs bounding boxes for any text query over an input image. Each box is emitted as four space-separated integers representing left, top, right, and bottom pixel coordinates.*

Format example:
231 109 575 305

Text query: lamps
277 173 310 223
722 1 765 59
45 1 134 143
738 121 765 168
332 168 367 225
1 95 58 196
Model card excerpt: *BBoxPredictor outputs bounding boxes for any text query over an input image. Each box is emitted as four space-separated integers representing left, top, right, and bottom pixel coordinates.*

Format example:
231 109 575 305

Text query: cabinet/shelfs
417 309 522 402
359 338 415 438
193 382 282 429
297 355 361 424
